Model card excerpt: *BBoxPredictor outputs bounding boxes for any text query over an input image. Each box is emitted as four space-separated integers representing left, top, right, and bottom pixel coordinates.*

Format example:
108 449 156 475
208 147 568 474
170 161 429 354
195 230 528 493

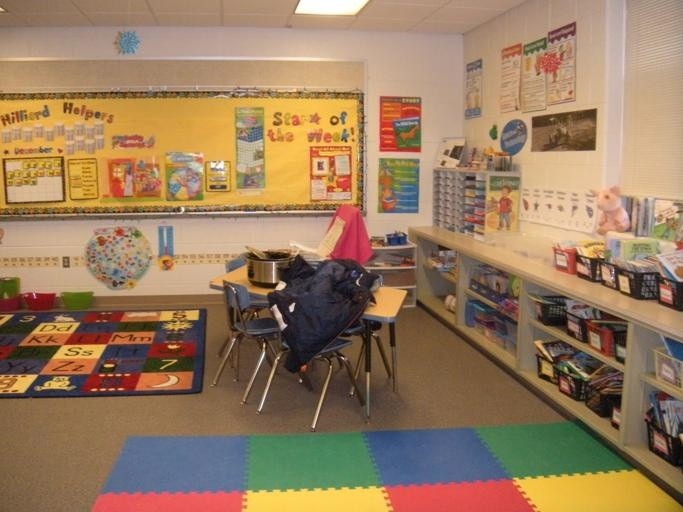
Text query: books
524 235 682 468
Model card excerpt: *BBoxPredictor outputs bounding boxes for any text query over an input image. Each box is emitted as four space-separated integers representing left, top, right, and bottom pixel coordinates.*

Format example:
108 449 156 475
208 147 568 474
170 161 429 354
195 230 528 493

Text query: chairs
257 291 368 434
211 282 291 406
214 259 278 360
342 274 395 401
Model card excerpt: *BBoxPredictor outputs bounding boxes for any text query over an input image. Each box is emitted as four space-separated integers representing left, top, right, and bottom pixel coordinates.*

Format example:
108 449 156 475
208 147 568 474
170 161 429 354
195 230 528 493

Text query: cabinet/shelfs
460 254 522 369
417 235 460 324
433 167 519 236
520 278 630 442
626 321 683 491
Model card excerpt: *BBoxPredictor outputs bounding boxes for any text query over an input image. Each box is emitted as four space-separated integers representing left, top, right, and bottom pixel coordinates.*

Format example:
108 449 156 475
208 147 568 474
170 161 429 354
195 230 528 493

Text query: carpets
0 308 210 400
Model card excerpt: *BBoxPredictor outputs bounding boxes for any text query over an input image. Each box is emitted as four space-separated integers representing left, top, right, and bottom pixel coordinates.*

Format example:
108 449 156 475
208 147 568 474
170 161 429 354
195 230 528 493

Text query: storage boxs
0 276 96 312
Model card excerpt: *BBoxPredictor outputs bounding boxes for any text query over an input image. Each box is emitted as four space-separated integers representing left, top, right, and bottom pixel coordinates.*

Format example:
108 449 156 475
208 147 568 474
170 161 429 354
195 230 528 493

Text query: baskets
536 355 622 419
653 347 683 393
535 294 627 364
553 244 683 311
644 415 683 469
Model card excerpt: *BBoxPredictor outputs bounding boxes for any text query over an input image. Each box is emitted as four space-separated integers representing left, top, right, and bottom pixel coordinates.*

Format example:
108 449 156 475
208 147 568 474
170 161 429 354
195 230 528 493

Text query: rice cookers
245 252 292 287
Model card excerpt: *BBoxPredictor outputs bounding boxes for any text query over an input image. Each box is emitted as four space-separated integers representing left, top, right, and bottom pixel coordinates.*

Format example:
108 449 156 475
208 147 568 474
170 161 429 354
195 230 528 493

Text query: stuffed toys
596 185 631 234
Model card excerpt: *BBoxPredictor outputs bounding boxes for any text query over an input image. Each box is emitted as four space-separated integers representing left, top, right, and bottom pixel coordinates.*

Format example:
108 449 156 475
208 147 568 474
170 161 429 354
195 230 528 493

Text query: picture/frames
364 242 418 309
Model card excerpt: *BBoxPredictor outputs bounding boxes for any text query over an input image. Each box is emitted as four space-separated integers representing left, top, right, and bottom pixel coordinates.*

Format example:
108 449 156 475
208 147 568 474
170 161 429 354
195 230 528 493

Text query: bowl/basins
24 292 55 310
59 290 94 309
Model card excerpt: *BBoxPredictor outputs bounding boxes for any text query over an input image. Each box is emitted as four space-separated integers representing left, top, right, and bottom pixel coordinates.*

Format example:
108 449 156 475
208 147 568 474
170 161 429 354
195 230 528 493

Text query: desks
210 261 408 393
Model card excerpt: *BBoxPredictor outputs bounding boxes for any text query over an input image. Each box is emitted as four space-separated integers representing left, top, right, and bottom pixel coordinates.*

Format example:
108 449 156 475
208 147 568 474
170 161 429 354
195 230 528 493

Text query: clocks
114 27 141 55
